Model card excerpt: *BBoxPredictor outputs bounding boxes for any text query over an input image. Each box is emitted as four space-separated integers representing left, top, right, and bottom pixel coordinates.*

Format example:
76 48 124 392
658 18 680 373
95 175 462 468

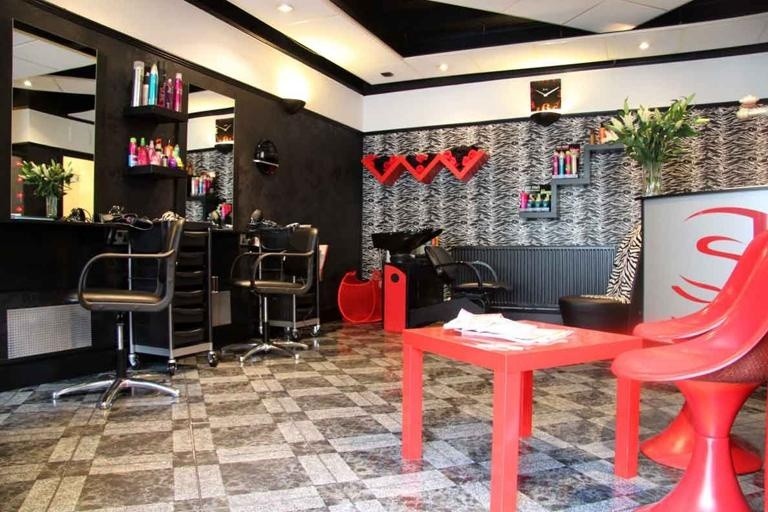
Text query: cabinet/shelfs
520 144 624 219
124 107 184 179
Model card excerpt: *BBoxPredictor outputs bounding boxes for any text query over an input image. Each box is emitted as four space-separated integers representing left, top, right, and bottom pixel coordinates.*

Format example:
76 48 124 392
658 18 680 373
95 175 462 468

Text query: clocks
531 79 561 111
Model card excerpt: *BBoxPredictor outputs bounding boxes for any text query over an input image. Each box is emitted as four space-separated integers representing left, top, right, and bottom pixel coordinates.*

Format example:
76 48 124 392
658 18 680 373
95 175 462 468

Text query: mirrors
10 17 98 220
186 82 236 230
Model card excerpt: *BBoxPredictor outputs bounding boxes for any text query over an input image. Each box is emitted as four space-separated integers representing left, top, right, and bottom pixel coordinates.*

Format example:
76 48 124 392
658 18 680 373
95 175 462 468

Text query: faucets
410 223 423 232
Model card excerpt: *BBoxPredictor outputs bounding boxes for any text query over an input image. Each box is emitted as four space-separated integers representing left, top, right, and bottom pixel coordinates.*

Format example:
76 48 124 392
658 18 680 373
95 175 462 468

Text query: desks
402 320 643 512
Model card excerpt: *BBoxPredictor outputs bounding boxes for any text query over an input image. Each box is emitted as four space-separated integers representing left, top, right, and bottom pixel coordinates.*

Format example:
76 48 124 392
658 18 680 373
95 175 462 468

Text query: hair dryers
248 208 280 232
106 205 153 244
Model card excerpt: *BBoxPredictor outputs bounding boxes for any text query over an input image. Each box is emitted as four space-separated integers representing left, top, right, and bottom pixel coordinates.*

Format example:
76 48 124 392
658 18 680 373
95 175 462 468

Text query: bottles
519 190 551 209
189 172 215 197
127 134 185 172
129 58 184 114
589 120 606 146
551 147 580 176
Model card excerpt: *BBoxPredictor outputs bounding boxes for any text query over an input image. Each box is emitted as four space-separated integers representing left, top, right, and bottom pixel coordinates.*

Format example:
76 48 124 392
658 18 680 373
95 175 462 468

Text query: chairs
52 219 185 410
559 220 643 330
611 246 768 512
633 230 768 475
220 228 319 362
424 246 514 312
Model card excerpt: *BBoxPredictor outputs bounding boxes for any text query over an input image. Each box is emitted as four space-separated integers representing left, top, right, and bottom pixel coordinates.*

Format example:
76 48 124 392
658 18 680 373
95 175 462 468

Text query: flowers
19 159 74 197
598 93 710 163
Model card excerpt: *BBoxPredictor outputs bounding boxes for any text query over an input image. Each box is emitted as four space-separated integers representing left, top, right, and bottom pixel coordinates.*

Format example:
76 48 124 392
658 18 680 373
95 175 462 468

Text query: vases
641 162 662 197
46 195 58 217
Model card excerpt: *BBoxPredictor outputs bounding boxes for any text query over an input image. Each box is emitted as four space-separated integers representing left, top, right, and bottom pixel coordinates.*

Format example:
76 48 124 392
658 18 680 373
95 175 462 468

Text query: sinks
371 228 443 253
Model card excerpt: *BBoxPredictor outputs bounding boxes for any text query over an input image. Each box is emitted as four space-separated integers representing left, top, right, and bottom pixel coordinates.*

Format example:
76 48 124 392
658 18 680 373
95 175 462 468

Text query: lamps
285 98 305 112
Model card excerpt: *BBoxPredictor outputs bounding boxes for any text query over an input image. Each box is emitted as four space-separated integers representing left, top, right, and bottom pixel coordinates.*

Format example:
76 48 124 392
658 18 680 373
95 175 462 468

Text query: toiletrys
552 148 578 175
519 189 551 207
125 57 186 170
189 169 216 198
